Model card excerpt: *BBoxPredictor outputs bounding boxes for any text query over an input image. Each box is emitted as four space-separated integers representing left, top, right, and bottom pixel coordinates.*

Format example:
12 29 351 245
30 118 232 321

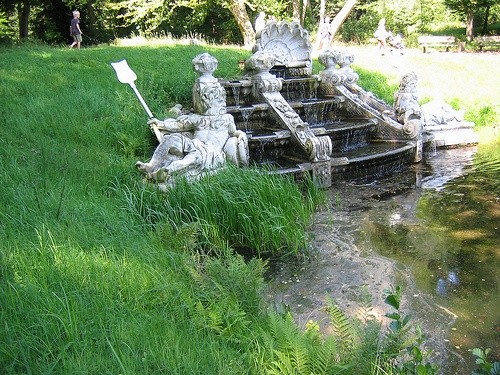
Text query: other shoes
68 45 72 49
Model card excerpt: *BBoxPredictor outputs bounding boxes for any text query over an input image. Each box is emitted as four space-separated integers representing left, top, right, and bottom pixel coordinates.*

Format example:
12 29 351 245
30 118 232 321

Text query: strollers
389 31 406 56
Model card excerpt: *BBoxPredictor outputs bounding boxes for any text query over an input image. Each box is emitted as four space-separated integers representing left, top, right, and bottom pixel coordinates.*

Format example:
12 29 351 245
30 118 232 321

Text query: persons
374 17 405 57
135 82 248 184
69 11 83 50
393 72 465 136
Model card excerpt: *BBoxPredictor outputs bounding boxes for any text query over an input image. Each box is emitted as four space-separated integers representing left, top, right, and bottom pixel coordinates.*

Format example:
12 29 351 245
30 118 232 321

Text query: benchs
418 35 459 53
474 36 500 51
